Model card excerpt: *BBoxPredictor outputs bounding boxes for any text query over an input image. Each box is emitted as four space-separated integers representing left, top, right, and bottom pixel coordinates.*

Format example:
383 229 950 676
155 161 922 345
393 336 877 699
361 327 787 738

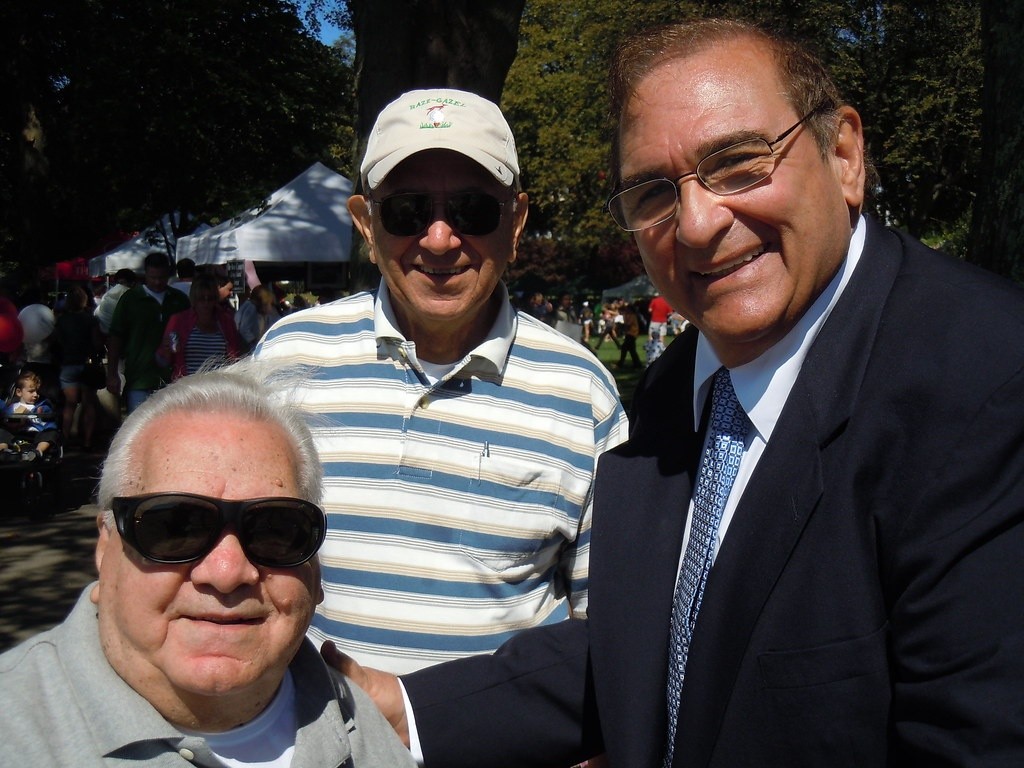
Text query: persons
250 19 1024 768
0 250 420 767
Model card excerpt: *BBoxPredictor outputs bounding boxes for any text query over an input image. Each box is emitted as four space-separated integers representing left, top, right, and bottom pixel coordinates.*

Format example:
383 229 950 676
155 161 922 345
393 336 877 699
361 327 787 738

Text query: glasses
104 492 328 568
607 100 841 233
367 191 516 239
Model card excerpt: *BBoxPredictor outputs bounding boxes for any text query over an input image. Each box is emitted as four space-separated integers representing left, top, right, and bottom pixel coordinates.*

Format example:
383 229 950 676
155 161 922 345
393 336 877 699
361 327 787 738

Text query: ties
661 366 749 768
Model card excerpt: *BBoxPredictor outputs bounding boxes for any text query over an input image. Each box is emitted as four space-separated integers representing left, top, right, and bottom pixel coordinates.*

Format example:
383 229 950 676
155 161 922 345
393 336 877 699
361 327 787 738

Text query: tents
602 275 660 303
56 161 354 316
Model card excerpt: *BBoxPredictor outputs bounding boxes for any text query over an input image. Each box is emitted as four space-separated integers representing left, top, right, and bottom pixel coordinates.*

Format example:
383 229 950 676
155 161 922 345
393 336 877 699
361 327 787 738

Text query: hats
359 89 520 196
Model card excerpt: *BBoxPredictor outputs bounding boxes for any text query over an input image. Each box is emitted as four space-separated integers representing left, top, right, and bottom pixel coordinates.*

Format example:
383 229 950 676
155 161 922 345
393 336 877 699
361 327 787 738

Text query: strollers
0 359 64 520
